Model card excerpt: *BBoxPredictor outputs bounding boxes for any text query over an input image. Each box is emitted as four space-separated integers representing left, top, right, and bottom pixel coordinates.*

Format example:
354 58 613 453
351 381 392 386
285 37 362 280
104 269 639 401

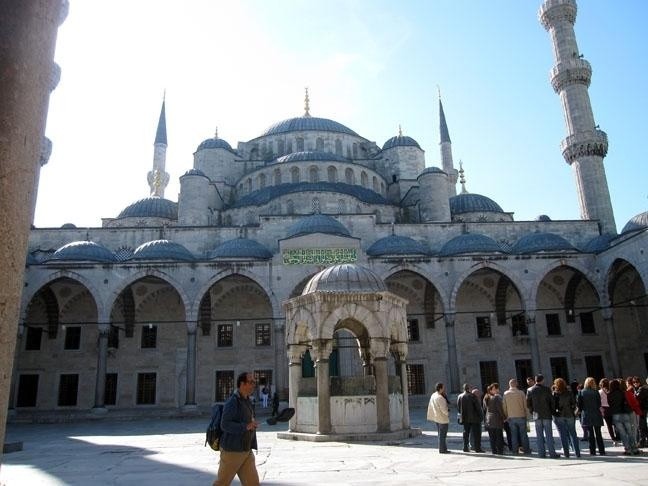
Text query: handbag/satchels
457 413 464 425
533 411 539 421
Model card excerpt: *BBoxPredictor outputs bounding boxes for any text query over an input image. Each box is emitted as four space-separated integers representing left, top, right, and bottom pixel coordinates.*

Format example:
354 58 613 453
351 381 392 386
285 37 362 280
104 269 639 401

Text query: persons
427 383 449 453
212 372 262 486
456 374 648 459
272 392 280 417
261 384 270 409
249 393 255 410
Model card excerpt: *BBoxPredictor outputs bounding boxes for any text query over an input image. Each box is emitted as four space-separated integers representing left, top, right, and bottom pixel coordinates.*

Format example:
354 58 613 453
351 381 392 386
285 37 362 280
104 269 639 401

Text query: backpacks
204 395 241 452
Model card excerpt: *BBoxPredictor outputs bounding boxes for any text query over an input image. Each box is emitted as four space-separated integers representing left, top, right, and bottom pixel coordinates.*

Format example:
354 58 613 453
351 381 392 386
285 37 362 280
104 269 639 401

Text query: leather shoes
438 434 646 459
